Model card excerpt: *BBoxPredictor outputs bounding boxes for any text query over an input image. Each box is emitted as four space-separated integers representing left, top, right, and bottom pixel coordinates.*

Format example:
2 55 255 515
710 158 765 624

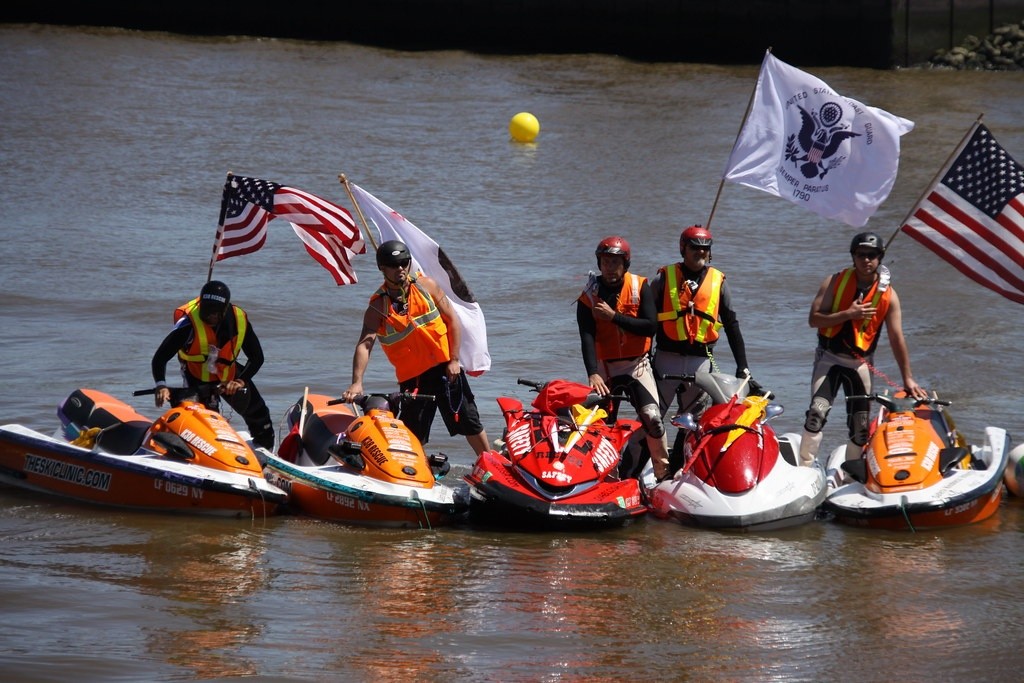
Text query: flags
210 175 364 287
345 182 490 376
725 53 916 228
901 123 1023 309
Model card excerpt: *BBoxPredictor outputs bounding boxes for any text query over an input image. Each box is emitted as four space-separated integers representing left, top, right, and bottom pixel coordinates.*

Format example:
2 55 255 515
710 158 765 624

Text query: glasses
686 243 710 252
380 258 409 269
853 251 879 261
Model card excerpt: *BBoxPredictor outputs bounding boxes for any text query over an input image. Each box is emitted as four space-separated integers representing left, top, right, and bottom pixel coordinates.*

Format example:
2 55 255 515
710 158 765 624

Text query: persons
619 226 755 482
797 231 929 484
577 236 675 482
149 282 275 452
342 240 491 457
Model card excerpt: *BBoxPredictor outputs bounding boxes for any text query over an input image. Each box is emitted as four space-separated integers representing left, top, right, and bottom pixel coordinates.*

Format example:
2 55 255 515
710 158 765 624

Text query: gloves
736 366 762 389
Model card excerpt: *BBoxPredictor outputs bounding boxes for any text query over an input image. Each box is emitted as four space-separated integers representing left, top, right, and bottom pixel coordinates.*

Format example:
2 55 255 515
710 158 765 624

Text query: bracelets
450 357 459 361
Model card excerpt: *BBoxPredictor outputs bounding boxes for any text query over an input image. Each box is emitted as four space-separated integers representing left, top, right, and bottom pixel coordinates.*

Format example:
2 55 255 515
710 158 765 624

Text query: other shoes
655 474 671 483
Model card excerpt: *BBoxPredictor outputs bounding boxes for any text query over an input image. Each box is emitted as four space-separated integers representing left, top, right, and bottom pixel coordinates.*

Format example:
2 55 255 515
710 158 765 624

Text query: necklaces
447 372 464 423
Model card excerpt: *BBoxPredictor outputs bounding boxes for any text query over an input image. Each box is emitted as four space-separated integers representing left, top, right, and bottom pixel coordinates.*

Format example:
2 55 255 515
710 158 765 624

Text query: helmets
595 236 631 271
376 240 411 271
850 232 884 259
680 225 713 254
201 281 230 306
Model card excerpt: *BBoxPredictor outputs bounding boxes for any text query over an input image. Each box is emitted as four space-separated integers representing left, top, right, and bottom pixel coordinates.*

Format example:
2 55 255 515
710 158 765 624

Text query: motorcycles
455 378 649 530
0 387 289 519
827 391 1008 532
640 372 828 532
235 391 456 527
